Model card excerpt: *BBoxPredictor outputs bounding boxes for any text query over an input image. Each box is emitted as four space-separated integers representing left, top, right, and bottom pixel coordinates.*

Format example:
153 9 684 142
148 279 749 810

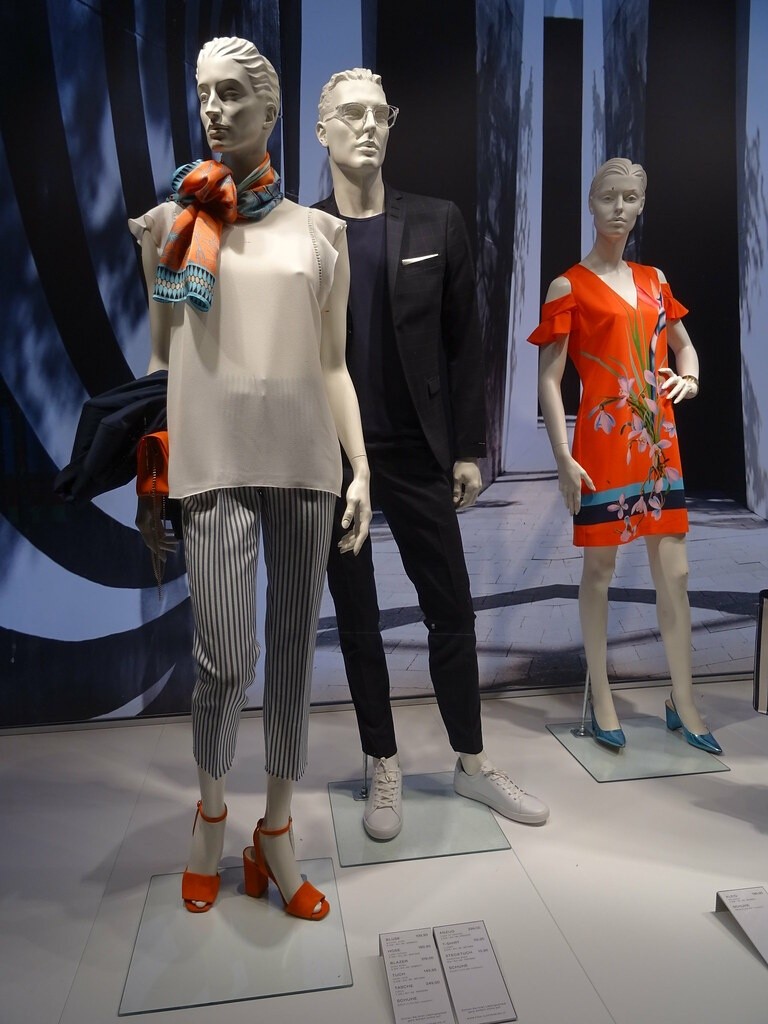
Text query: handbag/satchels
136 430 171 498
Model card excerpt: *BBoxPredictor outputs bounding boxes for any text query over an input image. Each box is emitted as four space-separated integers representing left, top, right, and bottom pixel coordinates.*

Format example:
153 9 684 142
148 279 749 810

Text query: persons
526 158 723 755
310 68 550 839
128 36 373 920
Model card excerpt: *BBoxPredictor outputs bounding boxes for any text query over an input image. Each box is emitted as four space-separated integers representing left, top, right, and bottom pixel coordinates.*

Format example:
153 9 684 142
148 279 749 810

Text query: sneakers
363 757 403 840
454 757 549 824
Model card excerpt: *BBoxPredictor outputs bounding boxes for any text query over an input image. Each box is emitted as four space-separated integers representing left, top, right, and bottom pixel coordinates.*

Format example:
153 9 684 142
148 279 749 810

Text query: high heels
242 818 330 920
665 690 722 753
589 695 625 748
182 799 227 912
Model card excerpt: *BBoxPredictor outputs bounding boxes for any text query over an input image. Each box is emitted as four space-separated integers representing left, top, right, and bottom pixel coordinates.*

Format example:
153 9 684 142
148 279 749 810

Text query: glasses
323 102 399 129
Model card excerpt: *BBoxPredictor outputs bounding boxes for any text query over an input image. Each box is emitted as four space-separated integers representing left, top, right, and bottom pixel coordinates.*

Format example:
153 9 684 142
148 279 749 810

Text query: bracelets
682 376 699 386
